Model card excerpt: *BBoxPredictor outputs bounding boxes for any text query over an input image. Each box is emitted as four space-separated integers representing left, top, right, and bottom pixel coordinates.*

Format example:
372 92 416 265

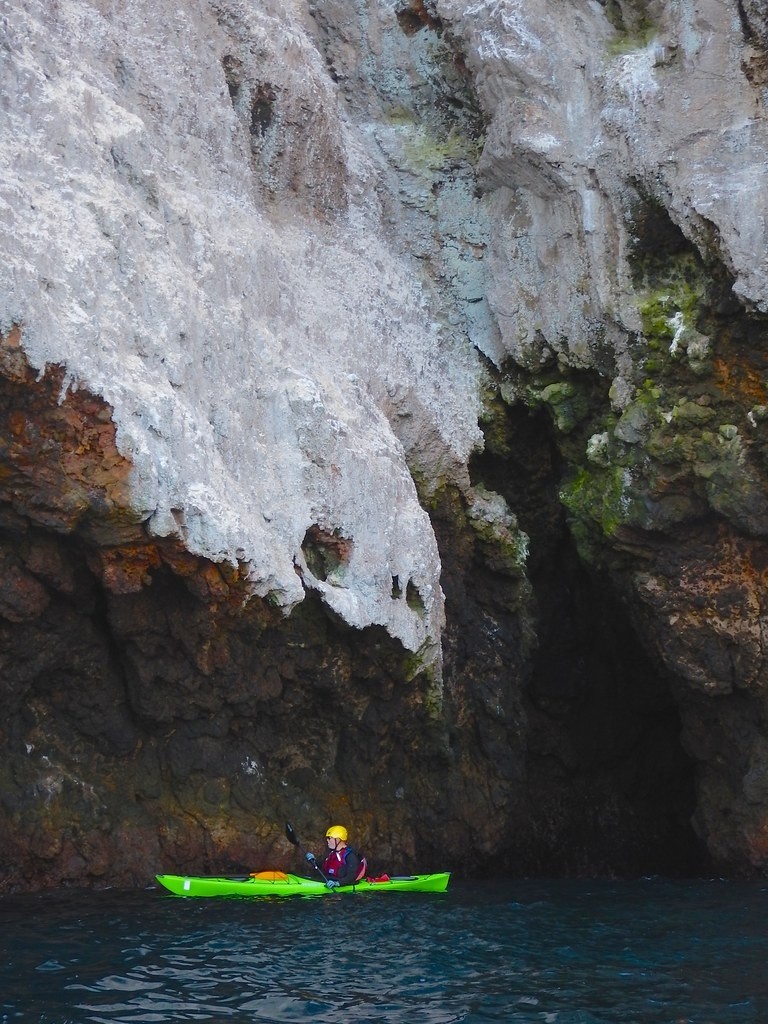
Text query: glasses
327 834 333 840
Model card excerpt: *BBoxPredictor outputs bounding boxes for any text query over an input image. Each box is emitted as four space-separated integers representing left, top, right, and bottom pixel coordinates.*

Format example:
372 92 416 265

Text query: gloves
304 851 316 863
324 880 340 888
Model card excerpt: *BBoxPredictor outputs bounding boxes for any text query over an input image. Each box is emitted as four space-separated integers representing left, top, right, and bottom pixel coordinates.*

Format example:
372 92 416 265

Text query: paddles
285 821 341 899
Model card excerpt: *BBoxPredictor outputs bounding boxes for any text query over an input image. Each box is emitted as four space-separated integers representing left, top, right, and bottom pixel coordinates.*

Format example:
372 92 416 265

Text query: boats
154 870 451 897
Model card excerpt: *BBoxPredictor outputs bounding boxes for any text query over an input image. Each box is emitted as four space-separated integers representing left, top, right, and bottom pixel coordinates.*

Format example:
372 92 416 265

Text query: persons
305 826 361 889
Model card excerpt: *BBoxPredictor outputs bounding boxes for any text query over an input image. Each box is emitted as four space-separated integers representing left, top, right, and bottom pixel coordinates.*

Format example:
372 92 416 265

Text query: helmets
326 826 348 841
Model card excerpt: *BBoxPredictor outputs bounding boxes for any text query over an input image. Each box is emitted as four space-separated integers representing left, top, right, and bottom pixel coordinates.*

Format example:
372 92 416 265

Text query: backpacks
344 848 367 883
